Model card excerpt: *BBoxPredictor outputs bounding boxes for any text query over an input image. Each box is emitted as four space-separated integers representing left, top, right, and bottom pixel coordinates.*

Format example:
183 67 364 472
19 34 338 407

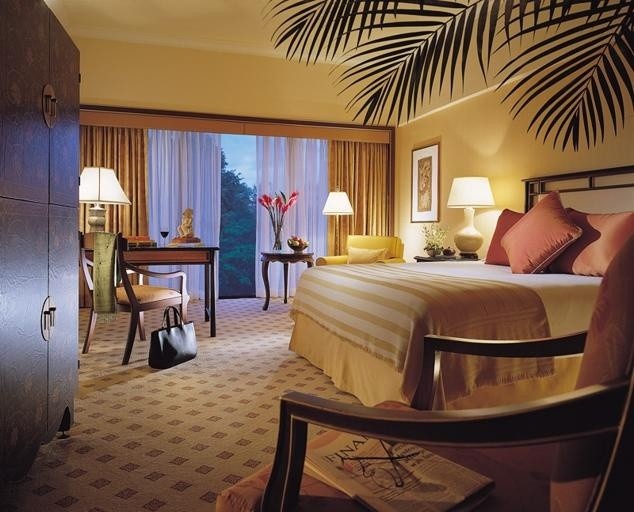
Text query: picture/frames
411 142 440 223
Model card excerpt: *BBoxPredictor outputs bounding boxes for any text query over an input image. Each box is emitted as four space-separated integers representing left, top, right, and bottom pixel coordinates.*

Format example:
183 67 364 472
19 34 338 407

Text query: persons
177 208 194 237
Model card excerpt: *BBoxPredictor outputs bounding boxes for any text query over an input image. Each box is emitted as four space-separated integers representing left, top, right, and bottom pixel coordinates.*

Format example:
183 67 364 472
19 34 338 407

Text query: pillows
347 246 387 264
542 207 634 278
499 190 584 274
484 209 526 266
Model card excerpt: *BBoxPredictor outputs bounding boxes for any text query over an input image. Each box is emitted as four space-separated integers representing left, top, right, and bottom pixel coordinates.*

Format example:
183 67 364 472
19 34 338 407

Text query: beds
288 165 634 409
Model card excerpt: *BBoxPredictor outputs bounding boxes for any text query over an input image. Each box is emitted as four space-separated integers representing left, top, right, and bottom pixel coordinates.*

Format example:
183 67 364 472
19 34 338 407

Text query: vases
271 225 284 252
426 247 441 258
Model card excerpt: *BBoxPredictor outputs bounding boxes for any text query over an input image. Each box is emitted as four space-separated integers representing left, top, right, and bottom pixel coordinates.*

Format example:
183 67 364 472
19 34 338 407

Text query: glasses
342 440 419 489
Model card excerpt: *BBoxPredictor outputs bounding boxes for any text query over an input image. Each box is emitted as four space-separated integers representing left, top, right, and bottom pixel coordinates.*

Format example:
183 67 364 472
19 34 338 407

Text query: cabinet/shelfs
0 1 80 491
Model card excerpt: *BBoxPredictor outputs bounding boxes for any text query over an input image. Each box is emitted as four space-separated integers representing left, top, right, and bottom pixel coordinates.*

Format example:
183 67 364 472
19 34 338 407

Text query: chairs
316 234 405 266
215 233 634 511
80 230 191 367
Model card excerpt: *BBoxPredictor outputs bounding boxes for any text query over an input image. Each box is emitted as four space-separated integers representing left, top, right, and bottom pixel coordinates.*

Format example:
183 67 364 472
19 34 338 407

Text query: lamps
78 166 133 232
447 176 496 260
321 191 355 255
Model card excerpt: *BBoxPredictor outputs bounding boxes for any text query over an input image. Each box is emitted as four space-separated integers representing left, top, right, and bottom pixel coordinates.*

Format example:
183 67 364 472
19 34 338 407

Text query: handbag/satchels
149 306 197 368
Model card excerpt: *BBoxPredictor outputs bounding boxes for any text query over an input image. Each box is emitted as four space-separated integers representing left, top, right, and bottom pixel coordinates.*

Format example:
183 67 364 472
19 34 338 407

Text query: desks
80 246 221 338
260 251 314 311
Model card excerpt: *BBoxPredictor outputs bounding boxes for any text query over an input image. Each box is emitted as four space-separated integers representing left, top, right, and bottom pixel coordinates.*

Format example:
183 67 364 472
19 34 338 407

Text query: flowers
256 191 299 221
424 222 446 247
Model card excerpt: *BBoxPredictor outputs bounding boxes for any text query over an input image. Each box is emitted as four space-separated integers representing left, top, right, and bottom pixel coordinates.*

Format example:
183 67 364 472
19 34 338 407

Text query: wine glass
160 227 170 247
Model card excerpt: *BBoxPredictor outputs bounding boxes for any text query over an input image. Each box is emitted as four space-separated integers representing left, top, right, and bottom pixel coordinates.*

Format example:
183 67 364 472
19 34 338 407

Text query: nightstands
413 256 481 263
